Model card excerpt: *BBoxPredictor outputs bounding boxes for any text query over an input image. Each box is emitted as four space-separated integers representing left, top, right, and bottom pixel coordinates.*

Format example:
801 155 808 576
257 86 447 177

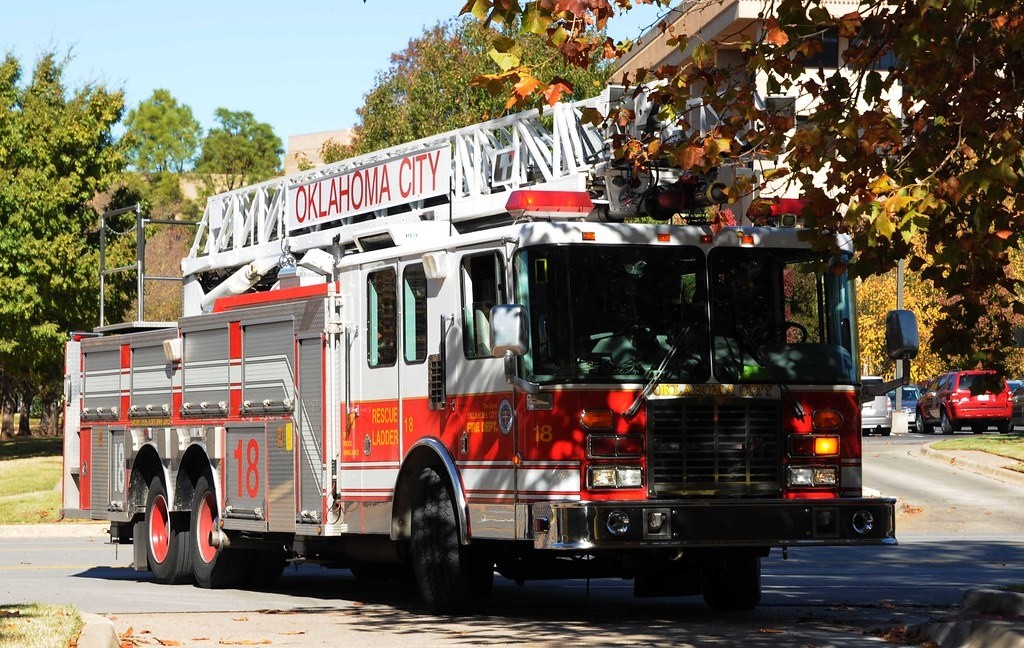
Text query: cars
887 385 921 433
1004 380 1024 430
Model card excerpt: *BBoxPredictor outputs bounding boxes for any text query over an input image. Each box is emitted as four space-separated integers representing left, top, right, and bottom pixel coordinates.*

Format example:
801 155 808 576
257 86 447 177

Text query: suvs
916 369 1012 434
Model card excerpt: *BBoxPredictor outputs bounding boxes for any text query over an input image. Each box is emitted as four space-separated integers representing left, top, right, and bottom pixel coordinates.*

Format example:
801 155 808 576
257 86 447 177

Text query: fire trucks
64 79 897 616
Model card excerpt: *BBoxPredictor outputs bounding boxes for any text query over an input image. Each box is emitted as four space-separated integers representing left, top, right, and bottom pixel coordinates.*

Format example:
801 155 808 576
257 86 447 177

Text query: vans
860 375 893 437
